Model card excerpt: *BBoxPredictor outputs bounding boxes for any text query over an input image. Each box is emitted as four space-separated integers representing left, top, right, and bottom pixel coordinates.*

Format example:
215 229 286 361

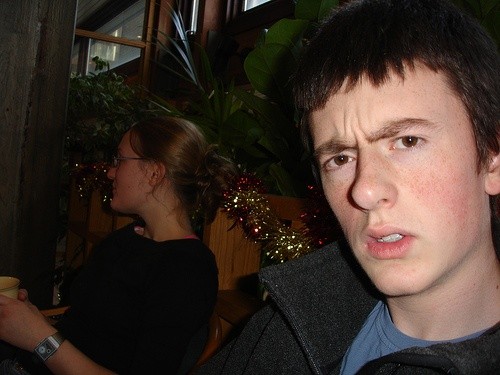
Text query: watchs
34 331 66 362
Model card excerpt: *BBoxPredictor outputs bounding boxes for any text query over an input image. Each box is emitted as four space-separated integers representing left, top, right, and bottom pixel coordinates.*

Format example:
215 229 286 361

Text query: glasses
113 155 148 167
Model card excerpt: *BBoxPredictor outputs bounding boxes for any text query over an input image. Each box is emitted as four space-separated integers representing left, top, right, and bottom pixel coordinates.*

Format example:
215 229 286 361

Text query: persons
184 0 500 375
0 114 241 375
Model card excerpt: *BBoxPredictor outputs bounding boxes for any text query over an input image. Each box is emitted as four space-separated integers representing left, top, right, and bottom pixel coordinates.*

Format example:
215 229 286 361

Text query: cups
0 276 20 300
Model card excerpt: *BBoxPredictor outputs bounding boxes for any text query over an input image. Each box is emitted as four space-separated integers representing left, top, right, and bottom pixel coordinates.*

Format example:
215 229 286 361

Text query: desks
200 289 254 364
